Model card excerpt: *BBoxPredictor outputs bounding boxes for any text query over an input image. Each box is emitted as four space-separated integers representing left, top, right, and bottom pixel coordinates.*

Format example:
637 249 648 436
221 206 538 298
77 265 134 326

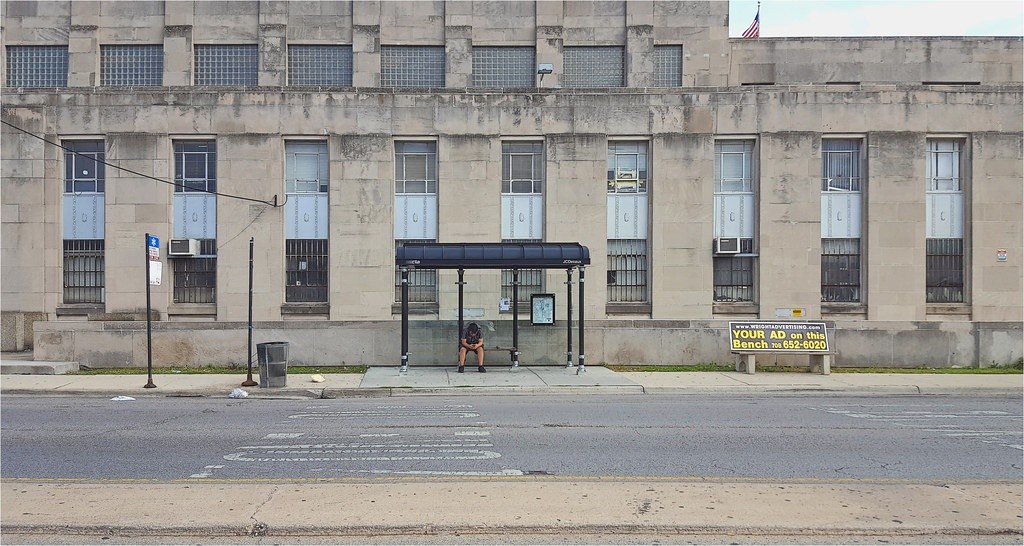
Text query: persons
459 323 486 372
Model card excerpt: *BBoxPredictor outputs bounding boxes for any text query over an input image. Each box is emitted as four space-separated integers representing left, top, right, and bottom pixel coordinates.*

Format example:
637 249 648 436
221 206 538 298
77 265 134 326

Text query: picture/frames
530 294 556 326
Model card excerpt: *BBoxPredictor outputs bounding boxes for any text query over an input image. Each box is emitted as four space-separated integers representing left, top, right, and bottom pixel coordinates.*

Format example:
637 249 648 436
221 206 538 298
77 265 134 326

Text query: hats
468 323 478 332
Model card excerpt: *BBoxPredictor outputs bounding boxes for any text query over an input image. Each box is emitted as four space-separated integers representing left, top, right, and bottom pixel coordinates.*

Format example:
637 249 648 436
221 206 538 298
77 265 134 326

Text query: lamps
537 63 554 87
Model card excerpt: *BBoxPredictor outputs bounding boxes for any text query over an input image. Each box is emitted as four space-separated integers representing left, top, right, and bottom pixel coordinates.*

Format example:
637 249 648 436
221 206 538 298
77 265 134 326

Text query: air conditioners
169 238 198 256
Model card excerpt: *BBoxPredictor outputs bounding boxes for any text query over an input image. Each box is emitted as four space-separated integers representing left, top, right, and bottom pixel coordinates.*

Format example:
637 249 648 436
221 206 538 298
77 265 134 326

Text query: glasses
471 332 476 334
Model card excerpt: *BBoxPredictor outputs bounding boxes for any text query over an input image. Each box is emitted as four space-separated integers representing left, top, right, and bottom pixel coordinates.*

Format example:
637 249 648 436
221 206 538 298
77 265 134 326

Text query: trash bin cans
255 342 290 387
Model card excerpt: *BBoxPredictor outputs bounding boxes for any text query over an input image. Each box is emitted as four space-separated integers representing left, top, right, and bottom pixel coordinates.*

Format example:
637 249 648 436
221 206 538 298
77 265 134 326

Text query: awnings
396 242 591 269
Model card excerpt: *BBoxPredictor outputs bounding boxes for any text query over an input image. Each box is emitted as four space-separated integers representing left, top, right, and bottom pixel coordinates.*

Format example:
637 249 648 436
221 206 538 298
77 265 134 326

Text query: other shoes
459 366 464 373
478 366 486 372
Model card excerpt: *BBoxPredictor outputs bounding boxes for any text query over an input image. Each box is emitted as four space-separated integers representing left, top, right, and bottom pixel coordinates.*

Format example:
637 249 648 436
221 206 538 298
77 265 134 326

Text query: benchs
730 350 839 375
457 346 518 372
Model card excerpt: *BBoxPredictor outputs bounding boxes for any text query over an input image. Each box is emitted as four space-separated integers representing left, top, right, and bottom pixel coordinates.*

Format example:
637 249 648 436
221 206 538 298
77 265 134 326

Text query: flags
742 11 760 38
